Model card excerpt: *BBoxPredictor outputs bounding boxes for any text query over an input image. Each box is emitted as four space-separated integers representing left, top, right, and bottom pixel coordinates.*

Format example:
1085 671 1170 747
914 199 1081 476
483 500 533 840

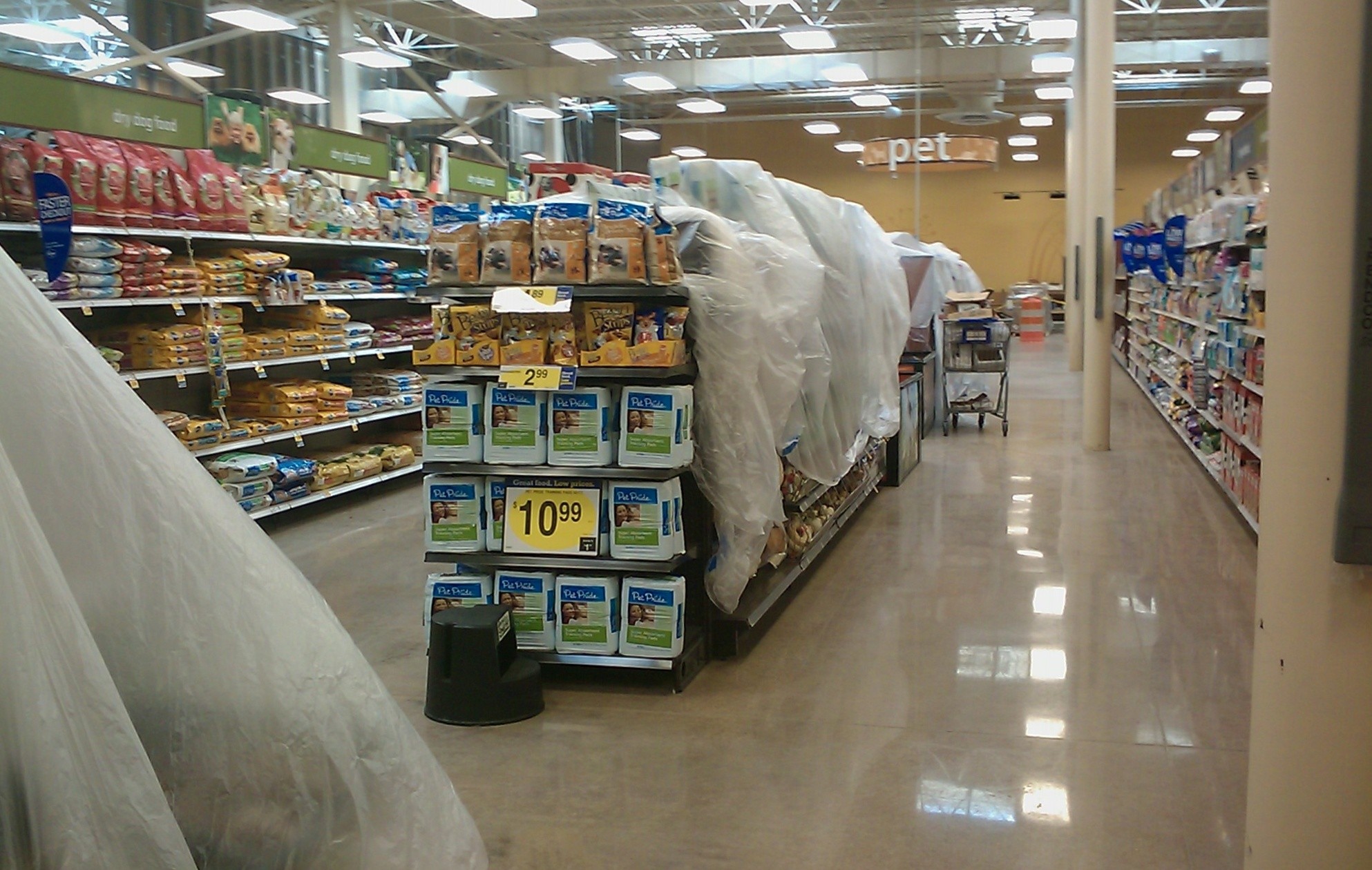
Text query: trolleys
939 312 1011 437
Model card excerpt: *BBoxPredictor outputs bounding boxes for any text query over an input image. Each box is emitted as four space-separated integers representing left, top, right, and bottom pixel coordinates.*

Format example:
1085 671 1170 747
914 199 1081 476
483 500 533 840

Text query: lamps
135 0 1277 163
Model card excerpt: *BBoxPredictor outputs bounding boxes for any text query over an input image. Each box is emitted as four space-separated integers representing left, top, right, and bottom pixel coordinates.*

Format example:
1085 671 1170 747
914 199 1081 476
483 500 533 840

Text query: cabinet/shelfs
0 121 1271 691
